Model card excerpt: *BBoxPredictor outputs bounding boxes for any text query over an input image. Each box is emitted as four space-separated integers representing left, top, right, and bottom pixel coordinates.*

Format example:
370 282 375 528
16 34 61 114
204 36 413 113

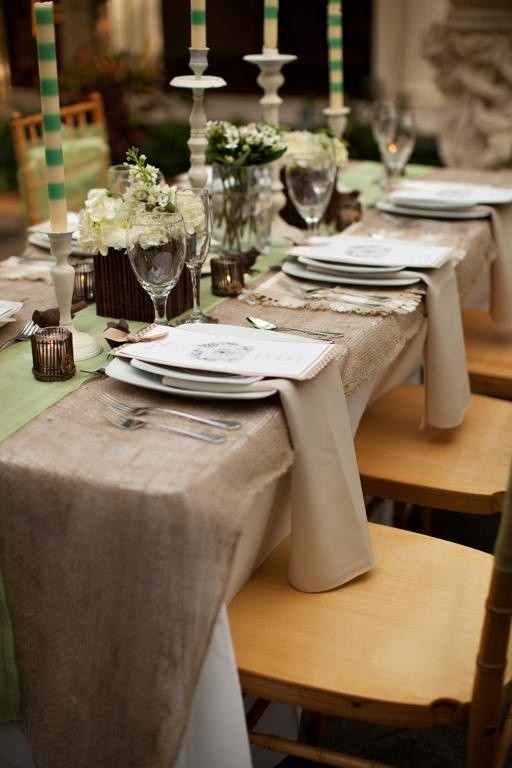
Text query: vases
204 160 274 255
281 174 338 227
93 233 194 326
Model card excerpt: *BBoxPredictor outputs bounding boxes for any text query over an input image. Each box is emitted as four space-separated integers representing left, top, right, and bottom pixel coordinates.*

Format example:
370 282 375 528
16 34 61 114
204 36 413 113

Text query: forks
101 403 228 447
94 386 245 430
0 319 38 353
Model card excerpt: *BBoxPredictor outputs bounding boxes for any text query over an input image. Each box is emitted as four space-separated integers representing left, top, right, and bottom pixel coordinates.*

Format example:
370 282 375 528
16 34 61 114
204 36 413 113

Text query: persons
419 1 512 173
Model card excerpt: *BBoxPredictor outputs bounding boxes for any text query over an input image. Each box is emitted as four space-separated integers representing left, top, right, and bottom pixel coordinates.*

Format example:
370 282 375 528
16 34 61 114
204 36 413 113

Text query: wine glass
127 210 187 336
286 150 333 243
169 187 219 327
310 135 337 238
371 97 420 181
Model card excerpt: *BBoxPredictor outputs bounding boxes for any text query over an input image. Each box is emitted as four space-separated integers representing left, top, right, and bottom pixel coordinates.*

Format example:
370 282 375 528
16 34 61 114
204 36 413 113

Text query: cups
104 163 129 196
72 261 95 305
30 324 78 383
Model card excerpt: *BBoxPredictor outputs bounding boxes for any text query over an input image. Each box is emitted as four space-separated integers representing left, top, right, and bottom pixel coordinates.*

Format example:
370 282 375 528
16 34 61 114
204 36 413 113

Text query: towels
159 353 382 596
306 247 477 434
425 204 512 323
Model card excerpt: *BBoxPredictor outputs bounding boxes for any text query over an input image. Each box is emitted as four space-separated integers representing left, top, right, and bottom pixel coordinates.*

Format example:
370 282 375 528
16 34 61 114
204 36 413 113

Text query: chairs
8 89 117 239
218 465 512 767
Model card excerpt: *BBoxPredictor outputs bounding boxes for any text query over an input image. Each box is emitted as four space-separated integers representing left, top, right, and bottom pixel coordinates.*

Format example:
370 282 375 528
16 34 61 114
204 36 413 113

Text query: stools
348 374 512 523
429 302 512 397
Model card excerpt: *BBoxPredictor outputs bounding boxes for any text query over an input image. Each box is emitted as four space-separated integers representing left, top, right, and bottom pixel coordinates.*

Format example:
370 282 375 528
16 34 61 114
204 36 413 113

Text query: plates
0 298 26 330
28 208 85 256
379 180 511 222
103 323 324 402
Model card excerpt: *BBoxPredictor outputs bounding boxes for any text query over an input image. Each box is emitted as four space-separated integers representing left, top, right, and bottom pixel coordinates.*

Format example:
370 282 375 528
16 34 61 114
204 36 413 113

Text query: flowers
201 111 286 261
278 129 350 171
76 143 208 259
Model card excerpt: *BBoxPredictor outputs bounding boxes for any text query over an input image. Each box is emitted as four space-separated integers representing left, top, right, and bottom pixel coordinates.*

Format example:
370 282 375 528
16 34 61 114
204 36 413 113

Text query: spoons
246 315 344 339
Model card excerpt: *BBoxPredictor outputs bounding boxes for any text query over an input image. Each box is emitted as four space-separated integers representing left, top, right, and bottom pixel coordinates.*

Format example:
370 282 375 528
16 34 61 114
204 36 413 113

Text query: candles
32 0 69 231
261 0 280 49
325 1 346 109
188 0 208 50
